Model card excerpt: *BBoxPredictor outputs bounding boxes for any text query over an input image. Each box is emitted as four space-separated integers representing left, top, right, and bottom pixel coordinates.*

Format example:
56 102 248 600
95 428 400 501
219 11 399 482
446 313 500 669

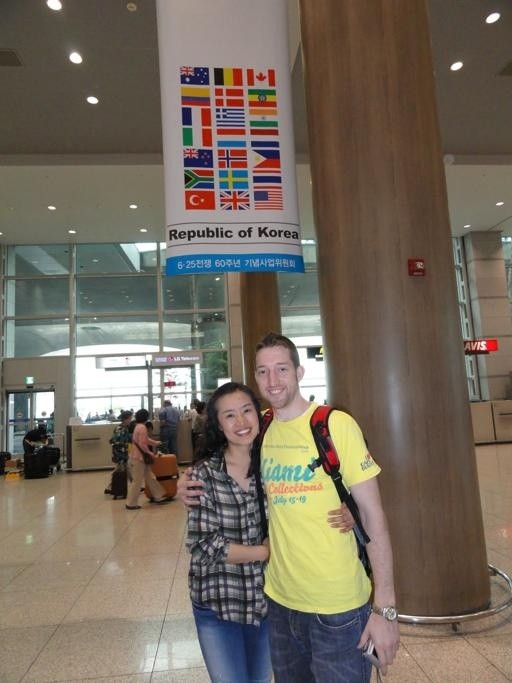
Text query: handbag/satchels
143 451 154 464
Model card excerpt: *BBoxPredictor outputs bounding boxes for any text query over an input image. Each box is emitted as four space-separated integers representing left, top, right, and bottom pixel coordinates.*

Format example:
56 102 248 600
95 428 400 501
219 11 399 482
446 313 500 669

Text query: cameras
361 638 381 668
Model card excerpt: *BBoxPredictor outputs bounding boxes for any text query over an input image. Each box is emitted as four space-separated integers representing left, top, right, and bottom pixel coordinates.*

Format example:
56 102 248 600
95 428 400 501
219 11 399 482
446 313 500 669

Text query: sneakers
104 488 112 495
140 487 145 492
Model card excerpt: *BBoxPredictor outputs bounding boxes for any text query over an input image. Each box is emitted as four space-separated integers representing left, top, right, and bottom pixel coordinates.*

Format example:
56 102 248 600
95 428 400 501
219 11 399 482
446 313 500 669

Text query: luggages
111 469 127 499
0 450 11 476
144 452 179 501
24 452 49 479
38 446 60 465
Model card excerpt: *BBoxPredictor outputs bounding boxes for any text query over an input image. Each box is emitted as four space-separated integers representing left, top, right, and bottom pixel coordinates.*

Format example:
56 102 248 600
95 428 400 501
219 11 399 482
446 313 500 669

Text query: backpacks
251 405 373 577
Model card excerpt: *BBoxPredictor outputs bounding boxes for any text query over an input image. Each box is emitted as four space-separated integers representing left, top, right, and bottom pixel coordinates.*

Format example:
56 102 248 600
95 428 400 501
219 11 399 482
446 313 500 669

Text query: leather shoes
126 503 142 510
153 495 169 503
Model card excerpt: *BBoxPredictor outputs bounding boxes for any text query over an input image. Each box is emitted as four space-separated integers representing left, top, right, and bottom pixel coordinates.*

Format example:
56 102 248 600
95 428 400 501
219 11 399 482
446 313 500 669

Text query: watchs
371 602 400 622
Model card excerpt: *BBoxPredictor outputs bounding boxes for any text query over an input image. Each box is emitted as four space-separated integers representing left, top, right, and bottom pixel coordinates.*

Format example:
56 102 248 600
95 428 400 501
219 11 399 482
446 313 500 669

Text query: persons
189 399 200 451
175 330 403 683
108 409 116 419
157 399 180 453
183 381 358 682
143 421 155 466
103 410 134 494
193 400 208 463
124 407 171 511
22 424 50 454
157 409 172 454
